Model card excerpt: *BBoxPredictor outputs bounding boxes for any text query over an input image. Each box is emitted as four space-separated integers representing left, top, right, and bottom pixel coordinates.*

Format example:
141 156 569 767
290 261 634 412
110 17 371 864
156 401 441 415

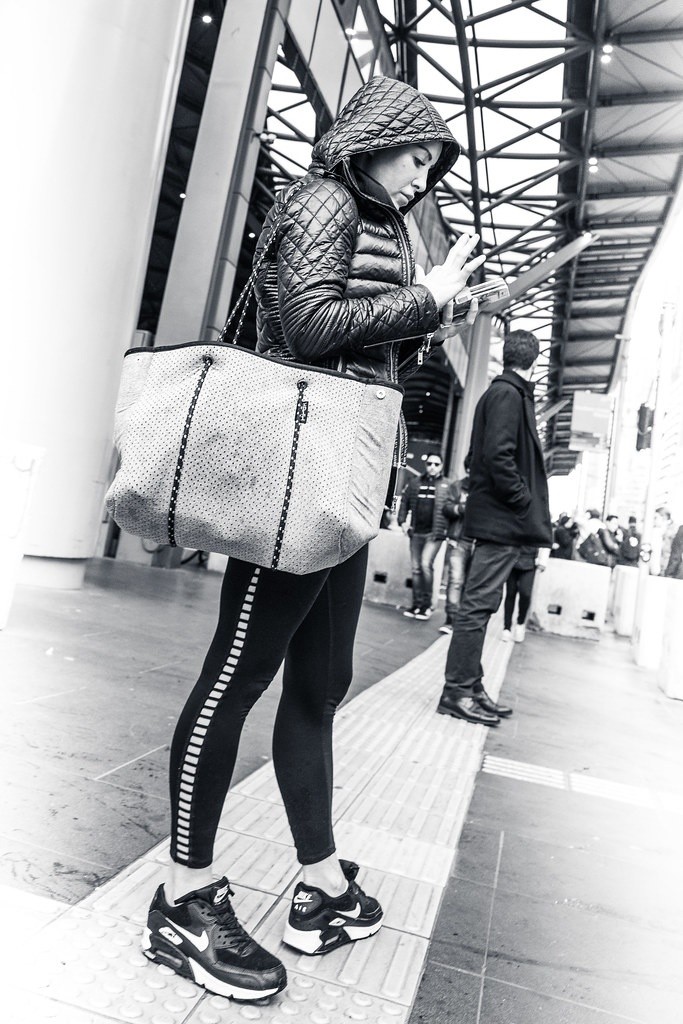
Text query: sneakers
138 876 287 1002
284 858 385 955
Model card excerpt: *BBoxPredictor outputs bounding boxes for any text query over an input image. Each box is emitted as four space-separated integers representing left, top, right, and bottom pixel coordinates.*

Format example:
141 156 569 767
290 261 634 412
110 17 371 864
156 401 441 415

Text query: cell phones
442 278 512 315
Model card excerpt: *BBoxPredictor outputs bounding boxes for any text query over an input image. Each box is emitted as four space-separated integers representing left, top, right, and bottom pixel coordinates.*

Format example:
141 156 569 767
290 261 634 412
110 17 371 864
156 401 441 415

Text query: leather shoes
438 688 514 725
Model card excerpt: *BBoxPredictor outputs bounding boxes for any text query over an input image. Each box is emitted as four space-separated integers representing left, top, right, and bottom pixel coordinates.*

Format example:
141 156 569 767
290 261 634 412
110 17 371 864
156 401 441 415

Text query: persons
139 78 490 1005
392 454 683 644
435 330 555 728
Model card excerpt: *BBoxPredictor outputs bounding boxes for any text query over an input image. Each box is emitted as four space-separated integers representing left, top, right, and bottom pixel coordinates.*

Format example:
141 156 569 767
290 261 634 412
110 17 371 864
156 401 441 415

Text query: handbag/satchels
101 168 404 578
578 534 609 564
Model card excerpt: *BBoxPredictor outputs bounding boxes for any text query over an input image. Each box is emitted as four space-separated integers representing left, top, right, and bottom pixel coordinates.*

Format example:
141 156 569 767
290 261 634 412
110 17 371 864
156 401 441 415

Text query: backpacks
620 532 640 559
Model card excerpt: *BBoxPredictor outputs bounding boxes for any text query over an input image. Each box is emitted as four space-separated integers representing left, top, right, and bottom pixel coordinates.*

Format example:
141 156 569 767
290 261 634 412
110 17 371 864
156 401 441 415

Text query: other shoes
512 623 526 642
402 605 432 620
501 630 511 642
438 623 454 634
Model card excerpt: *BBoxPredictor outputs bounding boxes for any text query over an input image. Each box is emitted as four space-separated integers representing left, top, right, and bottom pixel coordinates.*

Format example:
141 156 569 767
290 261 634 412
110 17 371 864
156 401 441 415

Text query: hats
560 516 569 525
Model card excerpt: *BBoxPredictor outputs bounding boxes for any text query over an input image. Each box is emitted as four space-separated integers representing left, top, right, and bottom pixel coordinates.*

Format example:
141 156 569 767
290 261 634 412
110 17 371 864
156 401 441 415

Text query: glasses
426 462 440 466
